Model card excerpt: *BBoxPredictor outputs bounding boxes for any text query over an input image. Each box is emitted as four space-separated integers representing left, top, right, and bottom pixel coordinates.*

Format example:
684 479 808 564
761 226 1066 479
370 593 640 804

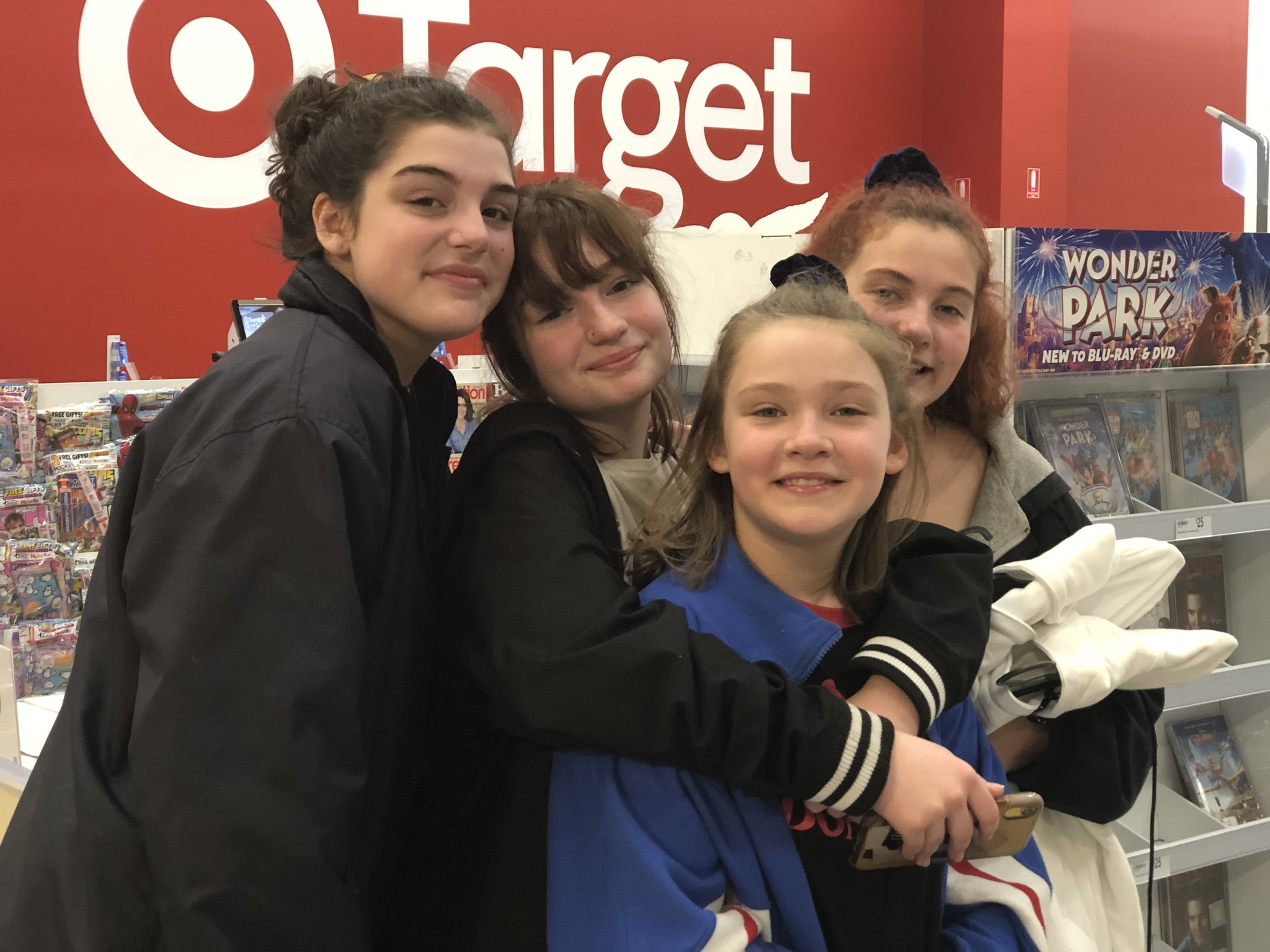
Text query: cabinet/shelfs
1004 356 1270 952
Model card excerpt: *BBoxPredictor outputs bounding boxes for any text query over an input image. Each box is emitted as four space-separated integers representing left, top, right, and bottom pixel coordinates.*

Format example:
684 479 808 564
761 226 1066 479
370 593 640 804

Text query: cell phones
846 791 1044 871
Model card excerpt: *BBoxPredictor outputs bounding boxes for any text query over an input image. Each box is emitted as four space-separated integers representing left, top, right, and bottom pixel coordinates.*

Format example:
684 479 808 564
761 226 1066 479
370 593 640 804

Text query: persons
802 187 1166 952
0 67 519 952
442 175 1005 952
541 250 1053 952
